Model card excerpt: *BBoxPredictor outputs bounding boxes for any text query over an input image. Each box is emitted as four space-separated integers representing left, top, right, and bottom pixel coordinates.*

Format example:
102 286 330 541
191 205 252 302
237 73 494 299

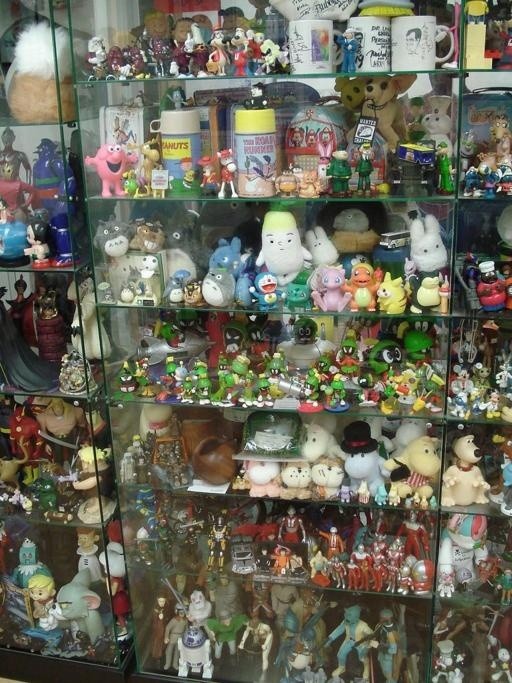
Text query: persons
28 527 121 630
1 0 131 671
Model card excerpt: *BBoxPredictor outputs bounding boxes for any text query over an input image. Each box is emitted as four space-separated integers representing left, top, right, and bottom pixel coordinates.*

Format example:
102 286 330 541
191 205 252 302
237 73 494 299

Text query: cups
348 17 390 71
391 15 453 69
288 19 344 73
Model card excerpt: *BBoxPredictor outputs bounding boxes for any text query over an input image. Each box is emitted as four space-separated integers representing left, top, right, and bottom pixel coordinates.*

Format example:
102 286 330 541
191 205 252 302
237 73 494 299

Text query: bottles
149 109 200 179
235 108 278 178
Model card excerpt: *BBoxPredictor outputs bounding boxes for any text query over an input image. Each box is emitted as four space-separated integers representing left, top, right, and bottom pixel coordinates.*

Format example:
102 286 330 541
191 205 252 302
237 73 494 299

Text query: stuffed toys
232 413 512 516
333 73 417 154
96 199 453 315
